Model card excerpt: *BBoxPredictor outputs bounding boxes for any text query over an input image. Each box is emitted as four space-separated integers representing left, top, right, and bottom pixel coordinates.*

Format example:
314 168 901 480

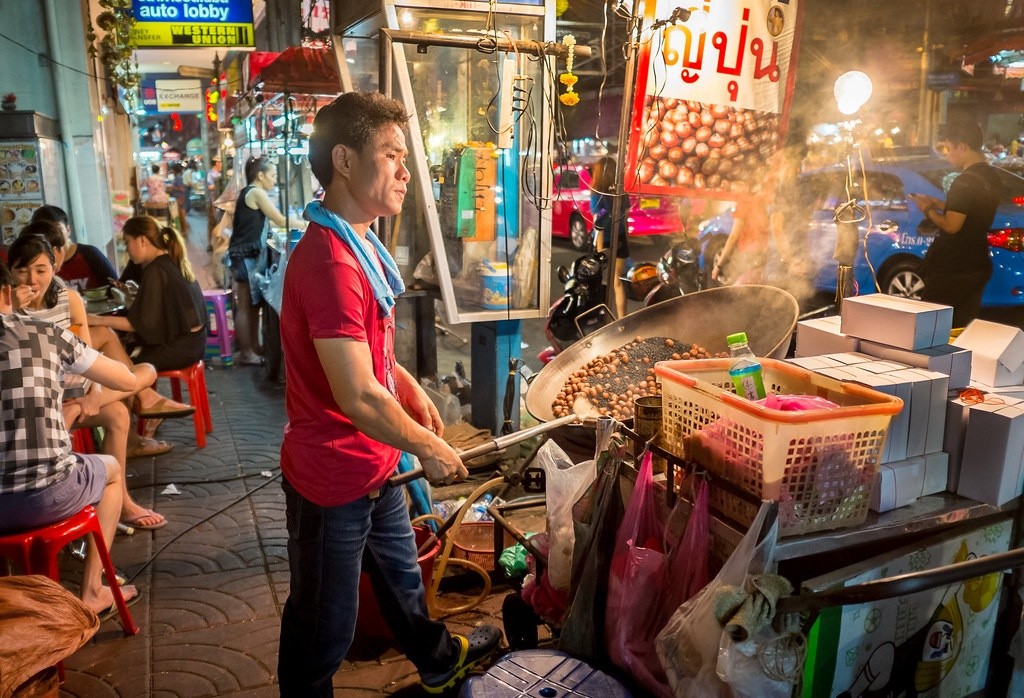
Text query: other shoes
206 244 213 251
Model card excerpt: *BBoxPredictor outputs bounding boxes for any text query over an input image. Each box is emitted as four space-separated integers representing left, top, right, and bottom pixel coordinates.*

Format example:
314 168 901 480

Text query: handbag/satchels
535 415 779 698
248 248 287 319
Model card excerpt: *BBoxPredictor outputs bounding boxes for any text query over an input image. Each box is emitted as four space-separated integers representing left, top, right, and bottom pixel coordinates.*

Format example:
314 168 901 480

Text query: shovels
388 396 602 488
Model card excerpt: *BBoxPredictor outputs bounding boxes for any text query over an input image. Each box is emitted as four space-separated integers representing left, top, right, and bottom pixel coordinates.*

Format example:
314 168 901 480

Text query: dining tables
77 291 122 312
63 351 102 397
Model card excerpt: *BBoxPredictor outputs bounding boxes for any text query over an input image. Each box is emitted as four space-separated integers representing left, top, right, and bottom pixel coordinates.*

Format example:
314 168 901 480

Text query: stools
0 504 139 683
200 289 238 365
144 363 213 449
459 649 630 698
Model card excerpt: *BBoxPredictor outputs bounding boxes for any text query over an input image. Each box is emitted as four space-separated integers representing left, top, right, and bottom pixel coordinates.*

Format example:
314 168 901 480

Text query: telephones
430 146 494 242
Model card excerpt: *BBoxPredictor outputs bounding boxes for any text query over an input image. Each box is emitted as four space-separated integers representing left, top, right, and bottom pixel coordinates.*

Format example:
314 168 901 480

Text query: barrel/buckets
477 257 512 310
356 525 441 637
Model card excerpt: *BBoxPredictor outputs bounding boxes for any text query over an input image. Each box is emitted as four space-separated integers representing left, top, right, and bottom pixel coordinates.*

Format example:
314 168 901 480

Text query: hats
211 154 221 162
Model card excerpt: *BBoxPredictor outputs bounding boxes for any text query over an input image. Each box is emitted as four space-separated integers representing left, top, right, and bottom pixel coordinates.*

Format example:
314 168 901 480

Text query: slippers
119 507 170 530
420 624 503 692
139 397 196 420
126 436 177 458
99 583 144 622
234 352 266 366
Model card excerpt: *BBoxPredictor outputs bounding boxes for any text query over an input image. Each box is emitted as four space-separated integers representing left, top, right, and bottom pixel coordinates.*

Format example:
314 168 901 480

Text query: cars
697 143 1024 307
551 161 684 250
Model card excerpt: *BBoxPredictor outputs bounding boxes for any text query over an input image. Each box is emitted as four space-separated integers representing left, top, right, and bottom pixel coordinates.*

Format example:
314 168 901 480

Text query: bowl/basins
81 287 106 299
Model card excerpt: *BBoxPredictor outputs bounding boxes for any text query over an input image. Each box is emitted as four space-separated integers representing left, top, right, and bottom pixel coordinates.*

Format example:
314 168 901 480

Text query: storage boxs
658 293 1024 539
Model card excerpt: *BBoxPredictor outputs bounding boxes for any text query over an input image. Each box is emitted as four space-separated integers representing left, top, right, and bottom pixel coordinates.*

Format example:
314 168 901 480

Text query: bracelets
925 205 938 218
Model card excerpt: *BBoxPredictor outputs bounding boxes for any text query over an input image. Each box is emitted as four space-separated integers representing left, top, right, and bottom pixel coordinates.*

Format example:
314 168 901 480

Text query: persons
18 206 210 457
991 133 1024 159
279 91 504 698
0 267 142 623
7 234 167 527
591 158 629 318
229 157 308 364
144 158 223 252
907 119 1000 328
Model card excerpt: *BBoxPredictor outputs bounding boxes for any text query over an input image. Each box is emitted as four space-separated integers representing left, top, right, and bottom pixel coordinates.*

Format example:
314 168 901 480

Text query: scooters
535 232 712 368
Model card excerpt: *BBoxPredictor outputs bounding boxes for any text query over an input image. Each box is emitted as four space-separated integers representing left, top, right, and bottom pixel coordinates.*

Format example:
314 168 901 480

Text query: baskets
655 354 906 536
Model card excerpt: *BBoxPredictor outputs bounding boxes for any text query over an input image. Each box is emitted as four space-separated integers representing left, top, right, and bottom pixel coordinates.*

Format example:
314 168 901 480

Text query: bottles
286 228 301 257
430 493 501 522
726 332 767 400
